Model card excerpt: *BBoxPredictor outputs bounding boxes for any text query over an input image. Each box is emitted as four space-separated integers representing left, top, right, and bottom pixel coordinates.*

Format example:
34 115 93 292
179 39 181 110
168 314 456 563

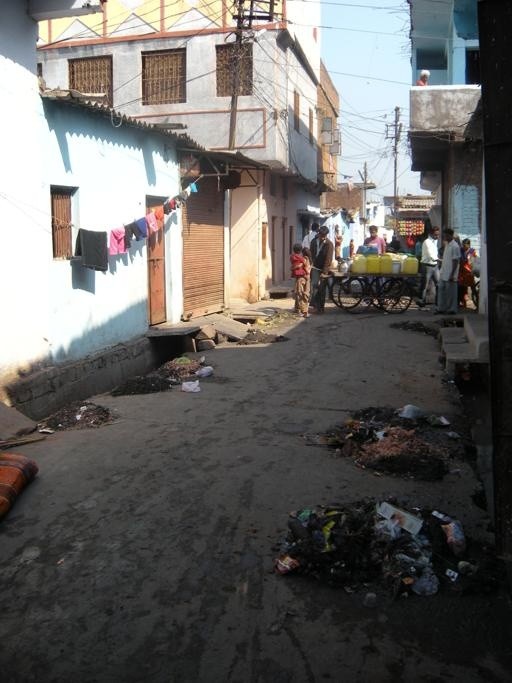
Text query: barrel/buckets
332 252 419 274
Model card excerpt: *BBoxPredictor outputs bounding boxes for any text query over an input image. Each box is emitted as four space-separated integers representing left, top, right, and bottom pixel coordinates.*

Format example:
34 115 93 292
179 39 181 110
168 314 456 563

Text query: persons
416 69 430 85
289 216 479 320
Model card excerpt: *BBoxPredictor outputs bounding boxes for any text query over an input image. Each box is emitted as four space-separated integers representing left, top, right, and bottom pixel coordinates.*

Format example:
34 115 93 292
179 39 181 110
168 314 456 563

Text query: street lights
392 106 402 207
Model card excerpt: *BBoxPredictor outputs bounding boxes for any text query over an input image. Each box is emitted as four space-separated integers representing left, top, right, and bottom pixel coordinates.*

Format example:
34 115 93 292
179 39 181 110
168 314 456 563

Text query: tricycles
330 273 479 314
